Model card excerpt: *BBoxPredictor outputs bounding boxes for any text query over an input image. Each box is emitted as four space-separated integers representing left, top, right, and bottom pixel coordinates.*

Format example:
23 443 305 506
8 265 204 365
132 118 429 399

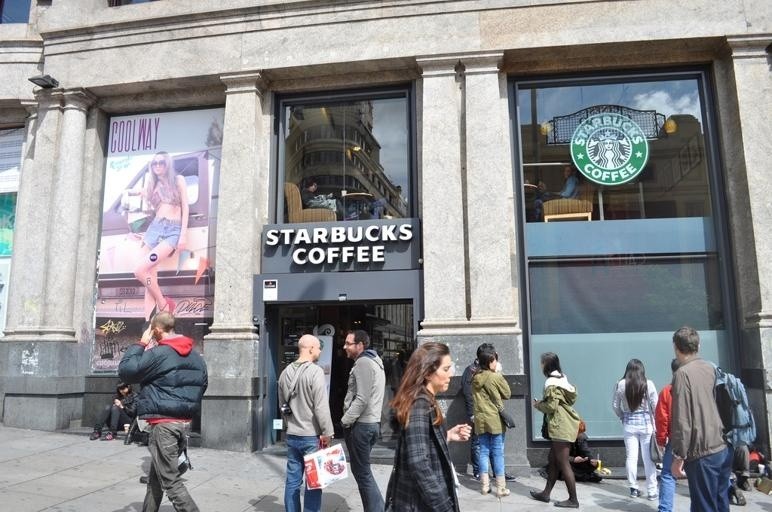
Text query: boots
496 477 510 497
479 474 491 494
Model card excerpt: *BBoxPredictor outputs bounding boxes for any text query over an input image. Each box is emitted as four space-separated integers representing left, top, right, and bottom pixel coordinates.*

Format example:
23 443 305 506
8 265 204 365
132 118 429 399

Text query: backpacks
713 365 759 448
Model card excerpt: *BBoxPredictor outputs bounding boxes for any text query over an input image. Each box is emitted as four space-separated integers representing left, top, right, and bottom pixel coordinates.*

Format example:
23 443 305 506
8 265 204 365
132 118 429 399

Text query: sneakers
630 488 639 498
89 428 102 441
105 429 117 441
647 494 657 501
473 474 480 481
738 479 753 491
505 474 516 482
734 488 747 506
140 474 151 484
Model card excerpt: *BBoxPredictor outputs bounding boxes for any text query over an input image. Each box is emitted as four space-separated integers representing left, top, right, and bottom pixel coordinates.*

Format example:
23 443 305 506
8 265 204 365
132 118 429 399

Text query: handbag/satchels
648 430 665 463
540 414 552 442
177 459 190 476
280 402 292 416
301 441 349 491
499 408 515 428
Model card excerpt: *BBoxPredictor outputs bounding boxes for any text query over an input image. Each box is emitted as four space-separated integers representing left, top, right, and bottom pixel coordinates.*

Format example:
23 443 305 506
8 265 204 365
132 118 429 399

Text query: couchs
542 182 596 222
285 183 336 224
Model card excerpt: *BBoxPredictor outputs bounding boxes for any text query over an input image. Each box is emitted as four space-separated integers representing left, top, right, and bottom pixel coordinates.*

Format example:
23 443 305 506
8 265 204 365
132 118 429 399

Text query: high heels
141 305 157 332
159 298 177 319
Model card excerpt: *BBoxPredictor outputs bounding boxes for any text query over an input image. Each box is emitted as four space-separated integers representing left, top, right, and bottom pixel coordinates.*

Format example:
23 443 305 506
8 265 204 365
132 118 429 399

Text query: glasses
151 159 165 166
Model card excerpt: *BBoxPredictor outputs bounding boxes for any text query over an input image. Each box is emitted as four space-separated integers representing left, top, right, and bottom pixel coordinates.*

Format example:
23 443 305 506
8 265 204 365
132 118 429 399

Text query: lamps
665 116 677 133
27 74 59 88
540 119 553 136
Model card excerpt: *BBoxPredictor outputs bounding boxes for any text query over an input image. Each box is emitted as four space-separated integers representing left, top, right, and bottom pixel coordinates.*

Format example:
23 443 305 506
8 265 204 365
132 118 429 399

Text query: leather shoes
529 490 550 503
555 499 580 509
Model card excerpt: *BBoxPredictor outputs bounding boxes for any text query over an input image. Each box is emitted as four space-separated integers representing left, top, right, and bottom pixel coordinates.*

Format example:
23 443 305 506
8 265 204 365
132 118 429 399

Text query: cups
124 424 130 434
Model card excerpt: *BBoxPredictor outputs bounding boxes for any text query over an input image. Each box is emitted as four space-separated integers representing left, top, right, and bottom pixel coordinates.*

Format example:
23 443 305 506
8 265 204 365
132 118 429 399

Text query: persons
90 378 138 441
533 166 580 221
340 329 386 512
133 149 191 316
471 348 510 497
277 334 335 512
118 310 207 512
655 358 682 512
301 174 401 222
383 342 472 512
529 352 580 508
125 415 149 446
611 358 659 501
461 343 518 482
728 443 772 506
670 326 757 512
545 418 604 483
